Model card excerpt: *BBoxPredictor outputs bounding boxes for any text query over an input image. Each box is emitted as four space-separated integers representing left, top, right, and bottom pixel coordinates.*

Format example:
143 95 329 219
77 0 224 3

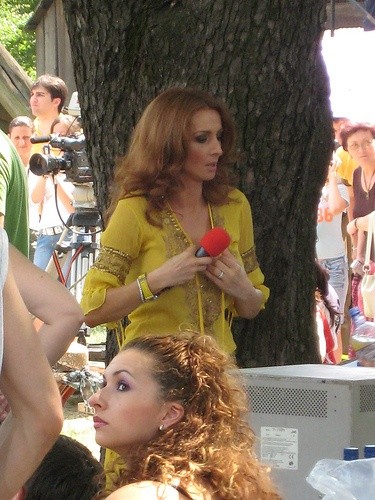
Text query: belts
41 227 62 235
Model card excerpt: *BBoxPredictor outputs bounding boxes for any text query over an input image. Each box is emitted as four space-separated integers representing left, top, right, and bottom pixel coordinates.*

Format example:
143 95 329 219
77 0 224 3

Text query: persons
313 116 375 365
0 73 106 500
79 88 269 490
88 335 283 500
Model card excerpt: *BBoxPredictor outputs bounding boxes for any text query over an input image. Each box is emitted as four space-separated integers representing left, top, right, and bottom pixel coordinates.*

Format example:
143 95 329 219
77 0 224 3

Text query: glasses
347 138 374 150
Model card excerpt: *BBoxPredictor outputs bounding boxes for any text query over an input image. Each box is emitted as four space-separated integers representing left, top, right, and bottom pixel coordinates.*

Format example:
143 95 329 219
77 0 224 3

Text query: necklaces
363 169 375 200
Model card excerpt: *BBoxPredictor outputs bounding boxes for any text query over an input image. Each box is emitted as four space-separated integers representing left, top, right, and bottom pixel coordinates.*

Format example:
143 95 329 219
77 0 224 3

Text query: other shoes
341 346 355 364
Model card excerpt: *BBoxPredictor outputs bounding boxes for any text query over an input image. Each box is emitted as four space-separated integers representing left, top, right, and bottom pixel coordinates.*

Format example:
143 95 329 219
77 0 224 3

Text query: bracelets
136 272 157 304
355 218 358 230
352 246 357 251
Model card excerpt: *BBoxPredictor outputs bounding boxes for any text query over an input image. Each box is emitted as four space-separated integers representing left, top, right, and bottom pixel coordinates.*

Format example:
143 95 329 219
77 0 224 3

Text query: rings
219 271 225 279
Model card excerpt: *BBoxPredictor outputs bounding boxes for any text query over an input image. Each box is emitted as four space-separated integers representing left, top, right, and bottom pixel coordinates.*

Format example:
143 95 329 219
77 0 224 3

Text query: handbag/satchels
360 274 374 318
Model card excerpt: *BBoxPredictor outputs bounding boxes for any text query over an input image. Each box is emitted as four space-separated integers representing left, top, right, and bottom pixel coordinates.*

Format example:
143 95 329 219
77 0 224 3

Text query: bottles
349 306 375 367
344 448 358 461
364 445 375 459
